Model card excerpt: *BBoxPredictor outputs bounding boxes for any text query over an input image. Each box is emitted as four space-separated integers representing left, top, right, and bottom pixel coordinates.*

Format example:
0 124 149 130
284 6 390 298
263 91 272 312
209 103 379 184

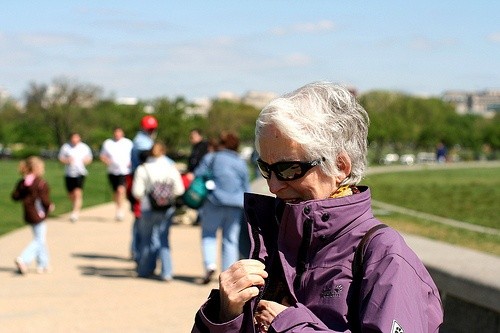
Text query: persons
127 139 187 282
125 113 162 222
98 122 136 223
184 125 212 227
57 124 95 224
8 155 57 275
184 128 251 285
187 80 446 333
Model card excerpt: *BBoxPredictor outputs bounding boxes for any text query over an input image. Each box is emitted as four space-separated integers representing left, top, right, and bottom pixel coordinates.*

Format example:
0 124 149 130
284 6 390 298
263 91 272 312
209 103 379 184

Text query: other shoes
203 269 215 284
14 256 26 274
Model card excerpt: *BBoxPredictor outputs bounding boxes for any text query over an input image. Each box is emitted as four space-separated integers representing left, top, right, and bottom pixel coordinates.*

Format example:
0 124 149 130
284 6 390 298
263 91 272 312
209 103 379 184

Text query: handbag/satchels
182 173 212 209
148 182 174 210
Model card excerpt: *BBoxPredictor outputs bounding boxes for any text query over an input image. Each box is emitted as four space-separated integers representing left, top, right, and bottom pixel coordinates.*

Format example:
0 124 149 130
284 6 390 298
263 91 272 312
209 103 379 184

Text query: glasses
256 156 325 181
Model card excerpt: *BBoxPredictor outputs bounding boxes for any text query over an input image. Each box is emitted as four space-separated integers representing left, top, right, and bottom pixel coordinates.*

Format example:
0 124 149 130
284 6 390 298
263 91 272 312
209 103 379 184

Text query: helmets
140 115 159 132
